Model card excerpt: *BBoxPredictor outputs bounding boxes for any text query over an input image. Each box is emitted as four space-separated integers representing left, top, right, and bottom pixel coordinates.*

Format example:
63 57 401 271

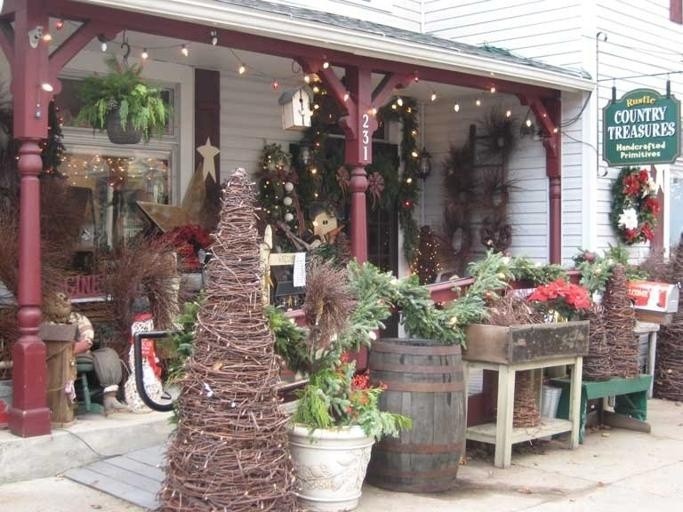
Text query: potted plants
74 58 176 147
346 244 514 495
167 260 415 511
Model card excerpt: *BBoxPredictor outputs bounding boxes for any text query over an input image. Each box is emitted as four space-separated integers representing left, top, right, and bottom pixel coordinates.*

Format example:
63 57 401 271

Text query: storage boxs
625 278 682 314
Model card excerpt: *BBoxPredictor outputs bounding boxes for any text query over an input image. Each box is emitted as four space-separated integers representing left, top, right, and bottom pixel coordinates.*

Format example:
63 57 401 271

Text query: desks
631 320 660 400
465 353 585 470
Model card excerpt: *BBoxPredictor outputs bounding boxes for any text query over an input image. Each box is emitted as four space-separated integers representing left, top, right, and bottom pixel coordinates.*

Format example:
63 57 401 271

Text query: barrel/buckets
369 339 468 493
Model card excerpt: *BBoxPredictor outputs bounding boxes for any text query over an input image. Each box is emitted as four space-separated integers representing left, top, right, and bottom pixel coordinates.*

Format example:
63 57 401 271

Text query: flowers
524 250 604 325
292 352 415 442
608 165 664 247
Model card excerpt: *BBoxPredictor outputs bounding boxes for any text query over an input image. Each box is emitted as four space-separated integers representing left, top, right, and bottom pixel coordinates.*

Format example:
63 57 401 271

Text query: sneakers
102 395 131 417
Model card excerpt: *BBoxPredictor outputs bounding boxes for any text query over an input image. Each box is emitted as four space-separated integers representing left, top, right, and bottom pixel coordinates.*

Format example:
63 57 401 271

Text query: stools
74 359 96 412
549 372 655 444
